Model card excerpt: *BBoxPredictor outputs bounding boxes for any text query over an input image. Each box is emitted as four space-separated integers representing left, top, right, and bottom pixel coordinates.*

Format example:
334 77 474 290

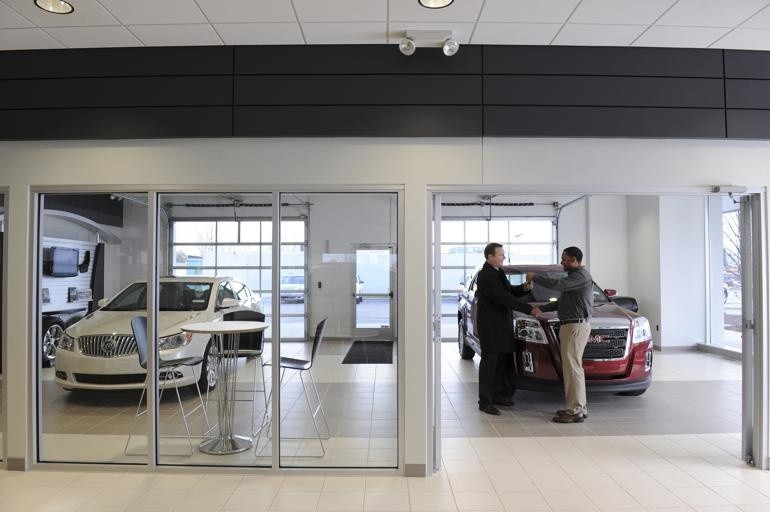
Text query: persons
526 246 595 424
475 242 541 416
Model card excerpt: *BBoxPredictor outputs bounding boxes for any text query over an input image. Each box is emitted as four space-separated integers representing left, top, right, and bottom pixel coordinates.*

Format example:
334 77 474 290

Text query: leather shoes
479 400 514 415
553 410 587 423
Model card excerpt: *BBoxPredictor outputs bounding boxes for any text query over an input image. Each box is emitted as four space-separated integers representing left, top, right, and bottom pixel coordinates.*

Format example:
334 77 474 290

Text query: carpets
340 340 394 365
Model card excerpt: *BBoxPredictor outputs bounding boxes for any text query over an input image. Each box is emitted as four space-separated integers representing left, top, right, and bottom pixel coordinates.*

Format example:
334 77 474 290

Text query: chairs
123 310 332 458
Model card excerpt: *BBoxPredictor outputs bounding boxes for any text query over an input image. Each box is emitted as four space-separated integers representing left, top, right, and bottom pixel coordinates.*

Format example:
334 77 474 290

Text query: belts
560 320 583 325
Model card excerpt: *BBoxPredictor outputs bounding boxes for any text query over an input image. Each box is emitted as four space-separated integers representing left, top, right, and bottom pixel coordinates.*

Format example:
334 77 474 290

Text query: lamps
398 30 460 57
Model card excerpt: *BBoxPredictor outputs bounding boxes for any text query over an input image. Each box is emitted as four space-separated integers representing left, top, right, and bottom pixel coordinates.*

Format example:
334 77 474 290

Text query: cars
53 276 264 395
280 273 365 303
458 265 653 396
